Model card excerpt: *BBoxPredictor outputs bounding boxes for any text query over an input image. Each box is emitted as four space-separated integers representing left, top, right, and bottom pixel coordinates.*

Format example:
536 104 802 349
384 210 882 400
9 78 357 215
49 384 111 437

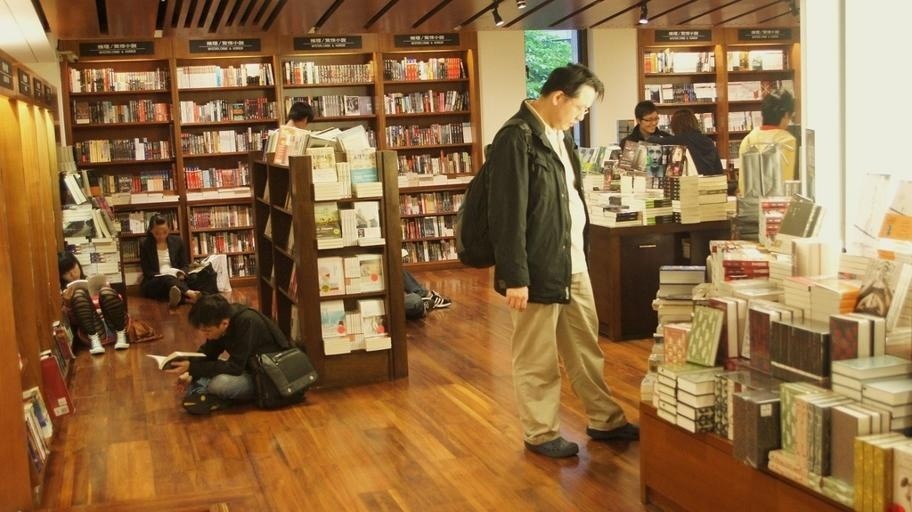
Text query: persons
487 62 640 459
140 213 215 304
165 293 293 413
404 270 453 320
56 251 130 355
620 90 798 197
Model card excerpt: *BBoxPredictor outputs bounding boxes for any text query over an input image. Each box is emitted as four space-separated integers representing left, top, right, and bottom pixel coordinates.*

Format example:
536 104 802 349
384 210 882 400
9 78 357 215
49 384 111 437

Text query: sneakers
168 286 181 309
181 391 234 415
524 437 579 458
420 289 452 309
585 422 641 440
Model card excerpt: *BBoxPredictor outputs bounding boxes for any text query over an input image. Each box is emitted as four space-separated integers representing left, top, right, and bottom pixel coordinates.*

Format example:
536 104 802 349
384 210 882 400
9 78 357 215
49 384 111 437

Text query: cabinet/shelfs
1 49 79 510
248 146 409 396
635 35 800 199
57 34 484 292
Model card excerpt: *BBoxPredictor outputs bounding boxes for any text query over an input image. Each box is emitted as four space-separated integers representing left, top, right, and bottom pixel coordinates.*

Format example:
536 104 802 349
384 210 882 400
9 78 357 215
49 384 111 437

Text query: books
384 58 474 262
154 261 211 279
640 176 911 511
645 50 789 171
176 63 278 278
284 60 393 356
71 67 179 263
144 352 206 371
578 141 686 228
66 271 105 297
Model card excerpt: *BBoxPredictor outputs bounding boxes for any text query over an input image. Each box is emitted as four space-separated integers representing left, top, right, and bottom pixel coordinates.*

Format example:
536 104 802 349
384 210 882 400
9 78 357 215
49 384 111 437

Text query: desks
636 400 858 510
578 212 734 342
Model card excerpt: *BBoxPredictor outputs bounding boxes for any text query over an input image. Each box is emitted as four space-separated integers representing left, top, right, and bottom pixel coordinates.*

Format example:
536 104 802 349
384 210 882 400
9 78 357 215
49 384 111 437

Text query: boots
114 329 130 350
87 333 106 354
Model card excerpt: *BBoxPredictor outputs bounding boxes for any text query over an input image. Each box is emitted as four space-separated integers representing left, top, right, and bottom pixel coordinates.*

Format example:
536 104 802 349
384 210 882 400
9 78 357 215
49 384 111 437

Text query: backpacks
455 118 533 269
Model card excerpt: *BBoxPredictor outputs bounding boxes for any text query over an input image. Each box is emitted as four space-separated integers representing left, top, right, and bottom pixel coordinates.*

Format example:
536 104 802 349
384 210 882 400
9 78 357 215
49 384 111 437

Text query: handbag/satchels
201 254 232 292
128 318 159 343
249 347 320 411
186 263 218 295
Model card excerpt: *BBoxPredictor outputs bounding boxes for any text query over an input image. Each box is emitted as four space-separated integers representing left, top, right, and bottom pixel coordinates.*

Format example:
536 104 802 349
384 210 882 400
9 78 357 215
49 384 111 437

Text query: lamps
451 0 527 36
635 0 649 27
